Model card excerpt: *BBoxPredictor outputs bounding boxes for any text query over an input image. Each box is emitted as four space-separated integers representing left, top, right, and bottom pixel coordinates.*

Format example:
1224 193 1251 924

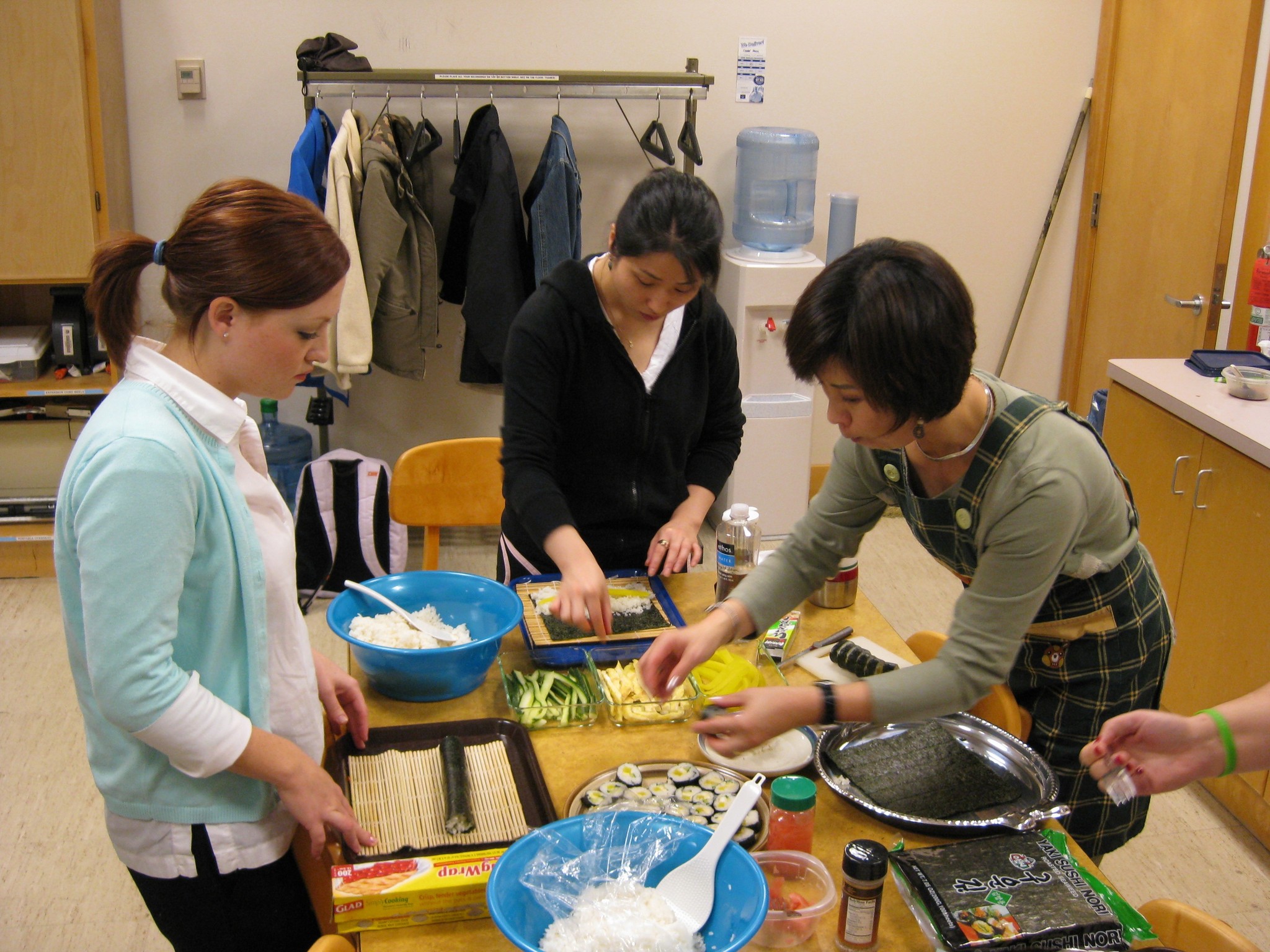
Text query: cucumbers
504 667 597 728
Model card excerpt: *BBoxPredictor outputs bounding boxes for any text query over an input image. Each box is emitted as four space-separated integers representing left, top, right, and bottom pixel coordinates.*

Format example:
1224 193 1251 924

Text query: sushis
581 762 762 853
829 639 901 678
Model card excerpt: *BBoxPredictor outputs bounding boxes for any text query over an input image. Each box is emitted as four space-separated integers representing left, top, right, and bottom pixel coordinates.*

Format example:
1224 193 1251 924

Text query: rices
348 603 480 649
539 880 734 952
530 582 655 619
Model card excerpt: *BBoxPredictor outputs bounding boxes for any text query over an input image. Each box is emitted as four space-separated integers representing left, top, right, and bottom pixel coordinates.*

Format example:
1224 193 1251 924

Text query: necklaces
599 258 643 348
915 374 994 460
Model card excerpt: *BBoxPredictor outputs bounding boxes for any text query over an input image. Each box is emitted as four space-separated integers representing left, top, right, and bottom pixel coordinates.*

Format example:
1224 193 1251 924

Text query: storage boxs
758 611 801 669
589 642 699 726
498 648 605 730
0 286 110 383
690 638 789 716
329 847 507 933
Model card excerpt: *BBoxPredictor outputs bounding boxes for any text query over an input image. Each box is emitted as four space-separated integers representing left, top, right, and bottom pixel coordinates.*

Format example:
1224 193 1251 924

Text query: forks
1101 767 1136 804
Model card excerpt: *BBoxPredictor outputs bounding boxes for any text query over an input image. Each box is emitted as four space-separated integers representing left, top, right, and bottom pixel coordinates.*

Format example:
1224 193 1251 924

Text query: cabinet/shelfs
1103 358 1270 852
0 0 141 578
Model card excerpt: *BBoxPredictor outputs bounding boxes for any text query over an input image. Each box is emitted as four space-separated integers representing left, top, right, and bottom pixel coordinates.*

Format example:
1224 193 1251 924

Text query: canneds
766 774 817 882
835 839 889 952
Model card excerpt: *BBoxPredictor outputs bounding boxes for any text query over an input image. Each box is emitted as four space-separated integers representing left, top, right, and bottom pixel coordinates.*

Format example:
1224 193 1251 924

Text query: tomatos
763 876 814 939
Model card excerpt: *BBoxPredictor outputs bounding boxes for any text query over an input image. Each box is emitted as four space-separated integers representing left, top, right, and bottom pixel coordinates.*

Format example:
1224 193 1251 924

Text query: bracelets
703 604 737 646
1191 708 1238 778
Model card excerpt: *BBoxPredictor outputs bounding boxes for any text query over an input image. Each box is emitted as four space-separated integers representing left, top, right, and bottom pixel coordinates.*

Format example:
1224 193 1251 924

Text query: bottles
1246 245 1270 354
733 127 819 252
764 775 816 881
255 398 313 515
835 839 888 952
716 502 761 603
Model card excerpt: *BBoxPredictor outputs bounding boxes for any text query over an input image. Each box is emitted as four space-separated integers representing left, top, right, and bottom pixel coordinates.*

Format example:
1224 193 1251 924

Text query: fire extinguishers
1246 244 1270 353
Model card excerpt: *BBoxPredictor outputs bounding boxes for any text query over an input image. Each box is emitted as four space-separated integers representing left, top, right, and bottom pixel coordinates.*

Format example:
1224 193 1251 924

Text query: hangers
549 92 565 166
640 92 703 166
370 90 402 175
484 91 503 171
331 90 359 155
453 90 461 164
293 90 328 152
406 91 442 164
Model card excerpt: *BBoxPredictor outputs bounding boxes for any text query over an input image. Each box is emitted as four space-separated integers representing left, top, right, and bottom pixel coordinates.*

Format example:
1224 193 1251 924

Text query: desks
348 572 1169 952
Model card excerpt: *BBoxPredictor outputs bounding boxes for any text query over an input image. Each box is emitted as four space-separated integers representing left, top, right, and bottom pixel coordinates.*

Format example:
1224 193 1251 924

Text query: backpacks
295 448 407 599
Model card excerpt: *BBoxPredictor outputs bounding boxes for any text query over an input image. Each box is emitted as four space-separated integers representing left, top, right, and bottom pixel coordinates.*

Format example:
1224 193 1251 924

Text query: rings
657 539 668 547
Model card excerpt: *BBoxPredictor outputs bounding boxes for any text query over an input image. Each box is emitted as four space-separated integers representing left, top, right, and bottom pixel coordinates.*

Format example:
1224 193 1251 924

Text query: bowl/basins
691 638 790 720
563 760 770 853
486 812 769 952
326 571 523 702
748 850 838 947
698 711 818 777
497 646 603 731
588 643 699 727
1221 366 1270 400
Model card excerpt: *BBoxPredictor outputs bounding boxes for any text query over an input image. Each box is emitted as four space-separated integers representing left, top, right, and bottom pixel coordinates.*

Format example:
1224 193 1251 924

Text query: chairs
1136 898 1262 952
390 437 505 571
905 631 1021 740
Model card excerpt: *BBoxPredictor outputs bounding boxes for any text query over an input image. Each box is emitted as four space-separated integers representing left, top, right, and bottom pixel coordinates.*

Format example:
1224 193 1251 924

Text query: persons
639 237 1174 869
1079 681 1269 807
54 180 377 952
498 168 747 642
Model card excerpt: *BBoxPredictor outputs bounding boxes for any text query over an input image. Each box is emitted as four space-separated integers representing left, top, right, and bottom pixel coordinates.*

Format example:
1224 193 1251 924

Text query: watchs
812 679 836 726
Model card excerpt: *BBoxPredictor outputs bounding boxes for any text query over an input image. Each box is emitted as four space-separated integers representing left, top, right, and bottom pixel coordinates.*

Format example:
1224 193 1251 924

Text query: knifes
776 626 854 667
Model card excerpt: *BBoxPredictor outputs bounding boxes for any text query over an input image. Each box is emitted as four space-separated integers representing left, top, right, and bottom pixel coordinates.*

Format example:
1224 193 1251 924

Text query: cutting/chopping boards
795 635 914 686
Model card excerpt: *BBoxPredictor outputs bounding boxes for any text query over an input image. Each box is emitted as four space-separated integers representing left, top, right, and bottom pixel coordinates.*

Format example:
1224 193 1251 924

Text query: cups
722 507 759 562
